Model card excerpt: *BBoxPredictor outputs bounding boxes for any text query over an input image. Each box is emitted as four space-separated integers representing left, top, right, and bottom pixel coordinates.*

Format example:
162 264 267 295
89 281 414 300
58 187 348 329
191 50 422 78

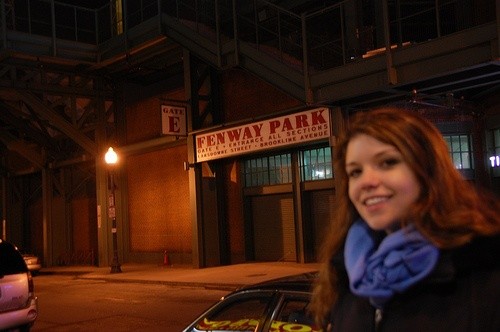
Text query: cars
19 253 39 274
182 269 335 332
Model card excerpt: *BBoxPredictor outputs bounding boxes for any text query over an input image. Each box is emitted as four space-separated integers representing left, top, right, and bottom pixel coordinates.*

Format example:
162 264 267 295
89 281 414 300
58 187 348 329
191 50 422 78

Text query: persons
306 106 500 331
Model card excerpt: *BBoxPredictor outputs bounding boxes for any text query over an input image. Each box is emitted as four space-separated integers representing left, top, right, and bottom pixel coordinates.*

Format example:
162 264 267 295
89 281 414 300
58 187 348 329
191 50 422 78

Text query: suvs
0 237 36 332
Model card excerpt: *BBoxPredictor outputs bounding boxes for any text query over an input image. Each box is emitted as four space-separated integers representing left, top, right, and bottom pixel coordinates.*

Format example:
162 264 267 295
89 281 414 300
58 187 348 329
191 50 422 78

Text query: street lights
104 147 123 273
